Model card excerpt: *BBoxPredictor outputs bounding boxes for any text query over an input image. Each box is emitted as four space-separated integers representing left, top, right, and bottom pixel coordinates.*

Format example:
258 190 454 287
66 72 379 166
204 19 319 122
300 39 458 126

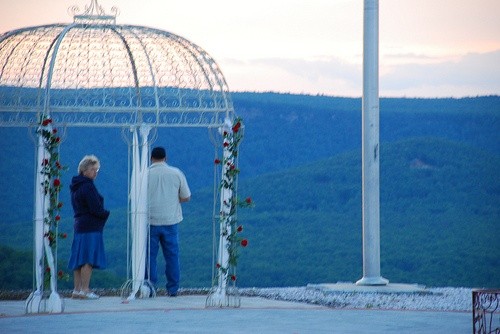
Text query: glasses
90 168 100 171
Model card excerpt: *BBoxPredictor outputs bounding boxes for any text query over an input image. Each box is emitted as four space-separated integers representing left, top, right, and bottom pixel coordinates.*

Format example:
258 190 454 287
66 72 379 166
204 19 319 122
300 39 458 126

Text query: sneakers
73 290 81 299
80 292 100 299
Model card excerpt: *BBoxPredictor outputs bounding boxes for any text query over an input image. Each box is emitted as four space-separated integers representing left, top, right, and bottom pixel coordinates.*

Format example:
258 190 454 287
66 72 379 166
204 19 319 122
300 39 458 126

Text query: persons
147 147 191 296
67 154 111 299
